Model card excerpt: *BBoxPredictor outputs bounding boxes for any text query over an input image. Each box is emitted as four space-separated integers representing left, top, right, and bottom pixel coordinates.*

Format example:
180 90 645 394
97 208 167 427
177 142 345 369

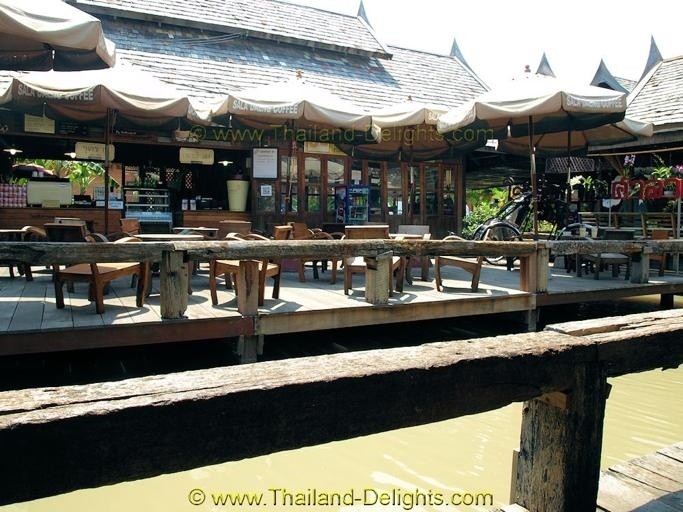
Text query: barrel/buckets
226 179 251 212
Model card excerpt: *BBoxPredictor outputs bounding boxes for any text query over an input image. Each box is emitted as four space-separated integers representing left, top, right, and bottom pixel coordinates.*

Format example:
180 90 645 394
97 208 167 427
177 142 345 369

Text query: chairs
574 226 673 277
0 215 496 314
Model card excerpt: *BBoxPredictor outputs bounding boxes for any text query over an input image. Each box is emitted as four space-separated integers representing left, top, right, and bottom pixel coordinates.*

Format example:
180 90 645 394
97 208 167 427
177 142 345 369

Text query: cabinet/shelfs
122 184 173 215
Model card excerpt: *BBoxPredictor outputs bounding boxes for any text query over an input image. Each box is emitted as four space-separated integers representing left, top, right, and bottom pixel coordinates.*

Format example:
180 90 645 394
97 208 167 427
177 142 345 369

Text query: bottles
337 196 344 223
348 194 366 206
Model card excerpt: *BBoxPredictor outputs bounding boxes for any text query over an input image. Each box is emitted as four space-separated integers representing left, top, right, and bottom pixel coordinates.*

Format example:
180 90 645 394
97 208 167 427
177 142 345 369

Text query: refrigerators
334 184 368 224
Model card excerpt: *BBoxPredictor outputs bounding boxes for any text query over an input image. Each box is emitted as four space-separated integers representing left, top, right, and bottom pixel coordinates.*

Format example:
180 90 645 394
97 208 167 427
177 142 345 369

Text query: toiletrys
0 183 27 208
